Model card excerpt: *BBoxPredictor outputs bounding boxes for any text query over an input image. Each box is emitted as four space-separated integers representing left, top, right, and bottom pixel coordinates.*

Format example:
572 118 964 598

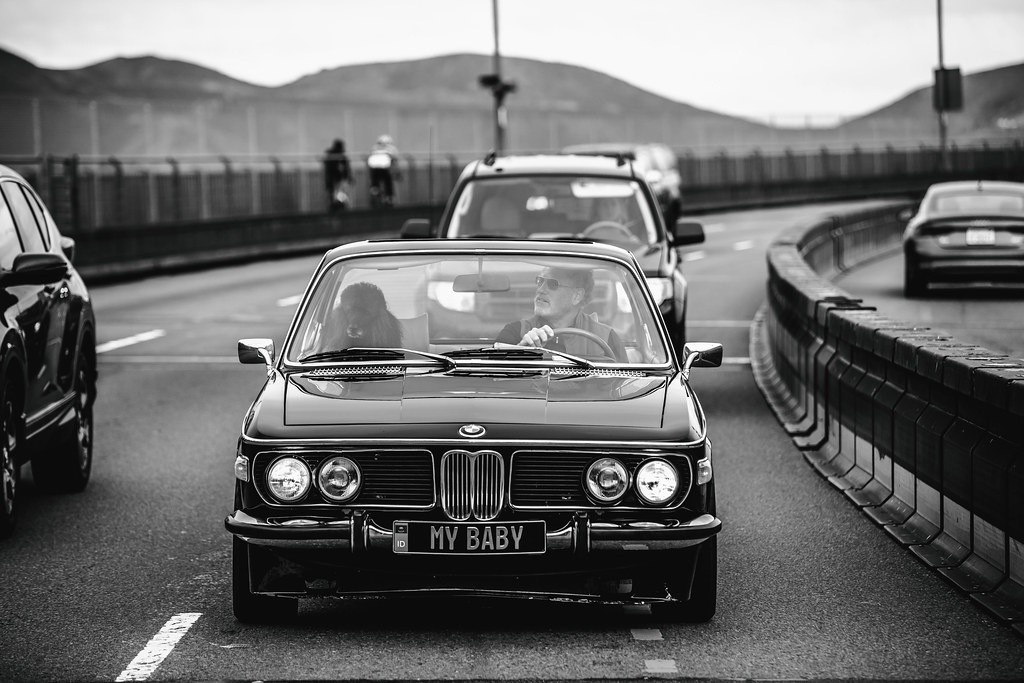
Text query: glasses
534 275 571 291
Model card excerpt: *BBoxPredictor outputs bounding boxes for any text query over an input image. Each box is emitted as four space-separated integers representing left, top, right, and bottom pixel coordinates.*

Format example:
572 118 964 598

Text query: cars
224 233 723 624
896 178 1024 298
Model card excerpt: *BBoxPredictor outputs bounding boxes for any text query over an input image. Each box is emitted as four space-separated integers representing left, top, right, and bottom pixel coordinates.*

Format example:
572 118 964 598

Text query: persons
323 139 355 217
492 265 629 364
571 197 642 244
368 133 404 211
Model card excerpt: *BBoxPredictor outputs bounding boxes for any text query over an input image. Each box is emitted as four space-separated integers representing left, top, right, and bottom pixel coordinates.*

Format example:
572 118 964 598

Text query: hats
376 134 393 144
325 140 344 153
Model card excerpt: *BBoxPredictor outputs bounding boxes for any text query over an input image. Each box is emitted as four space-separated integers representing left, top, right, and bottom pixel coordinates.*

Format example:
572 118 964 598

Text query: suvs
558 141 684 227
401 151 706 370
0 165 101 534
